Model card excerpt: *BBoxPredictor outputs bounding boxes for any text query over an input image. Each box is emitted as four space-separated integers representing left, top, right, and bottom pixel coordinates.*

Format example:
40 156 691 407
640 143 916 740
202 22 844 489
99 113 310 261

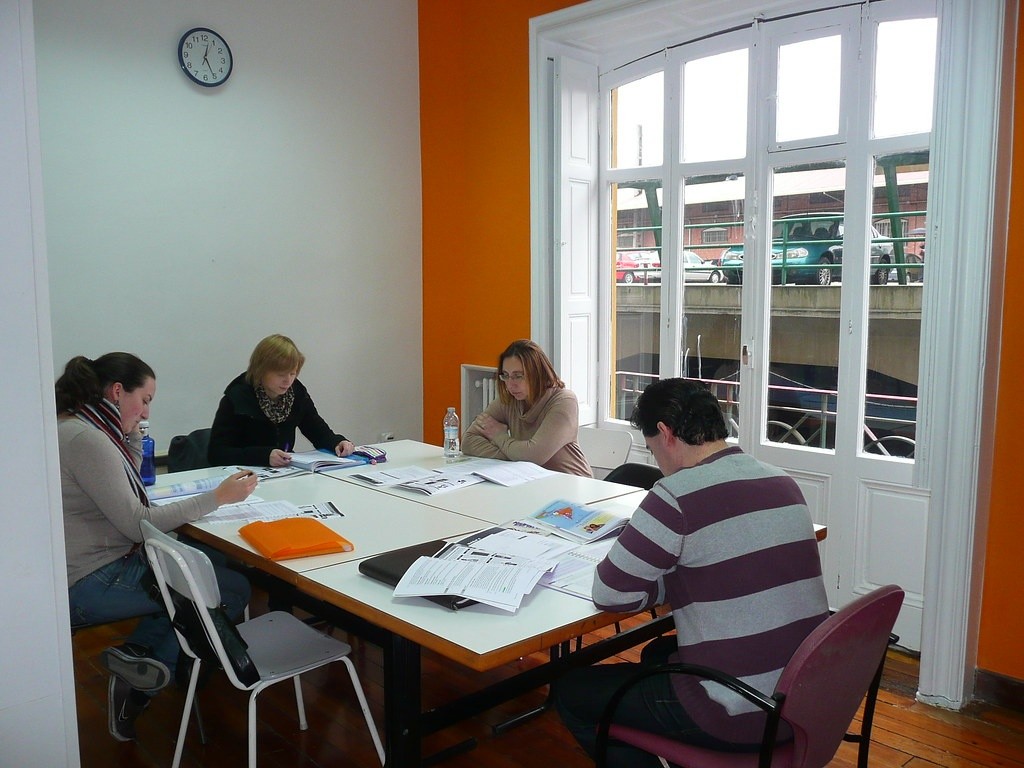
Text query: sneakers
108 675 151 742
100 644 171 692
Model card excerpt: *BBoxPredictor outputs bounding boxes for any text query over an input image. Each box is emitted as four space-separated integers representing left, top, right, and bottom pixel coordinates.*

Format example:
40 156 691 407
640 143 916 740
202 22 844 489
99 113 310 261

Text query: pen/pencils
283 443 290 461
236 467 254 474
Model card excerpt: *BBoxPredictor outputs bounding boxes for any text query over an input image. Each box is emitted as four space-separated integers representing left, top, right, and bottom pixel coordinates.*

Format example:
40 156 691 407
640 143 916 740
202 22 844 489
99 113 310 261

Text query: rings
482 424 488 428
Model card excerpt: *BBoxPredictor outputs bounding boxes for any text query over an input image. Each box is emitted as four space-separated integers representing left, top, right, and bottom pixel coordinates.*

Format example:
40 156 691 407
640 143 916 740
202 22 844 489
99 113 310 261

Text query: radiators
480 378 501 412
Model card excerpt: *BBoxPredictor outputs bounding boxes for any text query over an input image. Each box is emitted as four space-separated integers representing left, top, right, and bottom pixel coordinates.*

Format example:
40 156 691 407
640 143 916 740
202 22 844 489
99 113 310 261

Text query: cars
721 212 896 286
616 251 650 283
632 250 724 284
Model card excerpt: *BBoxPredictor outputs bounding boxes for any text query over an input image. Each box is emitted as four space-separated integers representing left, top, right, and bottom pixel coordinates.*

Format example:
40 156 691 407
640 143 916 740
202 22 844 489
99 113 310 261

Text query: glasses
499 373 525 381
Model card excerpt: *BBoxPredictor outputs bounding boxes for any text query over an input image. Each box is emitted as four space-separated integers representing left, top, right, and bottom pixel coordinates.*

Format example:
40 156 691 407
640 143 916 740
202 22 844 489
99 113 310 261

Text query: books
144 470 264 514
360 500 630 614
259 450 356 480
432 456 557 487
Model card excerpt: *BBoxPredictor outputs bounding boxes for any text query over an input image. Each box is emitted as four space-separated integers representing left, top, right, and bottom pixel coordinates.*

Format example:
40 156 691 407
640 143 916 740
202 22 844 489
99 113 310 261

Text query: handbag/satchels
238 518 354 561
172 599 261 688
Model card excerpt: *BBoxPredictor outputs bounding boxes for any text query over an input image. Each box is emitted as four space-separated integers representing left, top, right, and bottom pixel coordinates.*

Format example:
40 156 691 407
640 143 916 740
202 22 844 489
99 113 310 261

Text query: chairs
577 461 663 653
136 518 386 768
813 227 827 237
577 426 633 480
168 427 211 473
595 583 905 768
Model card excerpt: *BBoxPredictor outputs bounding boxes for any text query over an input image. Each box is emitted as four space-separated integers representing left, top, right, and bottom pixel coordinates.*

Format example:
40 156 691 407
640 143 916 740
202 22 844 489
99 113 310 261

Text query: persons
462 339 593 480
55 352 252 744
554 378 829 768
207 334 356 468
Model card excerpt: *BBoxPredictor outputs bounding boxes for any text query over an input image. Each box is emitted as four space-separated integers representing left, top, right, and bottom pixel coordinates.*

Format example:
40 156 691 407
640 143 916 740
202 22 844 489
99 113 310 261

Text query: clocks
178 27 233 86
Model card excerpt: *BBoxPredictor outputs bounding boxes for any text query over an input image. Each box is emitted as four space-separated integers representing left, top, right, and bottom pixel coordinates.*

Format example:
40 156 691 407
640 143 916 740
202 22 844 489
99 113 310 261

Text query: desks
147 440 830 768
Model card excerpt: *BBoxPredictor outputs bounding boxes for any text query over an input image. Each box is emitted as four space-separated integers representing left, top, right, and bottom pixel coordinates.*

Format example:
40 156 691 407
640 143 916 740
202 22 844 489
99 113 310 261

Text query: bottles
140 422 156 485
443 407 459 457
